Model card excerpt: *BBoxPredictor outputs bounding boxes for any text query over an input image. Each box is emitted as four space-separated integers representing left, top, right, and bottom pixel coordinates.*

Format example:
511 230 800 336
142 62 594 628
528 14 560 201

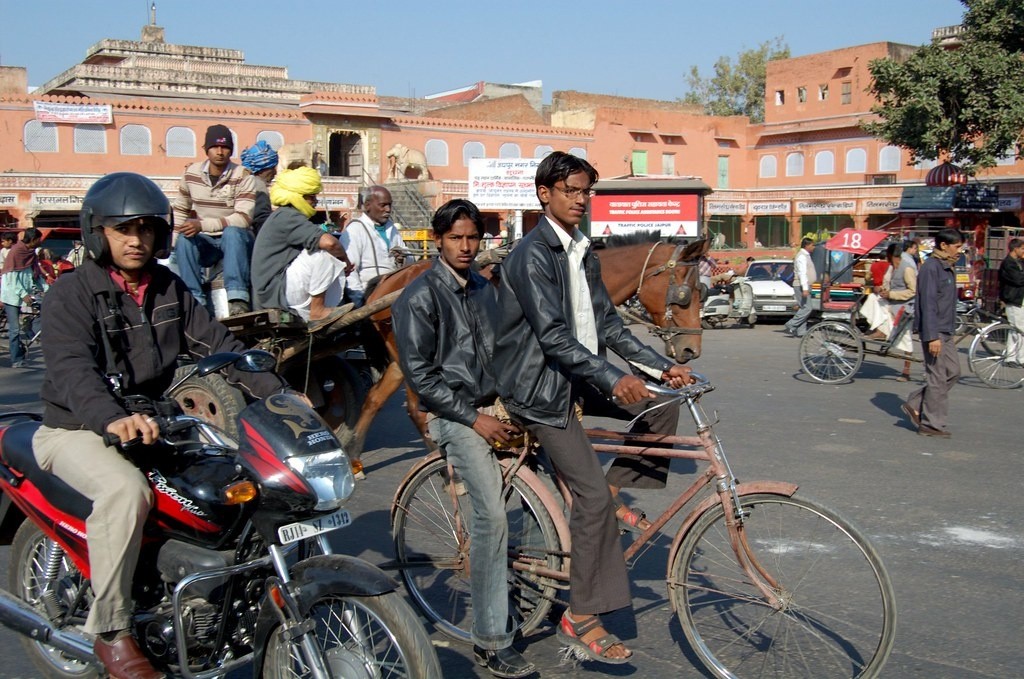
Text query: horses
348 225 713 460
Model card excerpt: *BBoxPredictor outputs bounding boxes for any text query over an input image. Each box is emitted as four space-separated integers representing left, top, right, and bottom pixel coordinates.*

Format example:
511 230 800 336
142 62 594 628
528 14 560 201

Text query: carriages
160 228 714 489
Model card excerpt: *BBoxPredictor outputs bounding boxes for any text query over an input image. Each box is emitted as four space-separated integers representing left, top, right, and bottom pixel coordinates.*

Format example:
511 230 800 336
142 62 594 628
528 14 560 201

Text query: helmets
79 172 174 261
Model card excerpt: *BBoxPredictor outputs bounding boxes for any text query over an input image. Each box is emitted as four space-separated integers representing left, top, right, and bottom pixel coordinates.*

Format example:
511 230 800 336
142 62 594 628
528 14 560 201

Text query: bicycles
367 372 898 679
797 287 1024 390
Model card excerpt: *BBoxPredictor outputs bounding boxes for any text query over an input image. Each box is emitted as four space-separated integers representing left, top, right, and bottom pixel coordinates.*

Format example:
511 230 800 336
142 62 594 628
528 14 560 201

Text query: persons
0 227 84 369
770 255 780 277
390 198 536 677
784 238 817 338
974 247 992 268
172 124 356 333
31 171 314 678
492 149 681 664
338 184 415 384
746 257 756 267
902 228 966 436
998 239 1024 369
864 240 921 382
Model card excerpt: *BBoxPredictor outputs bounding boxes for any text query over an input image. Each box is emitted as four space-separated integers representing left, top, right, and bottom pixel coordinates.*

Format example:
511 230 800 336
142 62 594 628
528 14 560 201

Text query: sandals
612 494 654 535
556 608 634 664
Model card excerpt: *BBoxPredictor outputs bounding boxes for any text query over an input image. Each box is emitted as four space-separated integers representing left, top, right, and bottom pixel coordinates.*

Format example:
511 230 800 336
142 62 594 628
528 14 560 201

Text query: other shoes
785 326 798 336
863 331 887 341
229 300 250 316
307 302 355 332
1002 360 1024 369
917 425 951 438
901 403 922 430
12 360 28 368
896 373 910 381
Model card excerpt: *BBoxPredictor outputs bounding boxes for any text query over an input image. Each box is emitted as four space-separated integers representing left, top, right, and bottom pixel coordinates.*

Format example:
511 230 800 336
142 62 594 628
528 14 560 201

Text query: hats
204 125 233 155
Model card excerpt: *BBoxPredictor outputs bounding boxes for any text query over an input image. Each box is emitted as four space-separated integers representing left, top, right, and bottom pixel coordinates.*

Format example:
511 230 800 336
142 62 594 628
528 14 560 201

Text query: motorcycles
0 348 444 678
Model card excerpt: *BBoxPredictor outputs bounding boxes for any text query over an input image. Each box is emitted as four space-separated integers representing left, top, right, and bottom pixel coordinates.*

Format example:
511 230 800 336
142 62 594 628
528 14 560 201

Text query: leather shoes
473 641 536 678
92 633 167 679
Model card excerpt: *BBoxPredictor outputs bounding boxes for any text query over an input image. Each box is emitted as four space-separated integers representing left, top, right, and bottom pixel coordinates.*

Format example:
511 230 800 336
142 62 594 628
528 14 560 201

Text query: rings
146 418 153 424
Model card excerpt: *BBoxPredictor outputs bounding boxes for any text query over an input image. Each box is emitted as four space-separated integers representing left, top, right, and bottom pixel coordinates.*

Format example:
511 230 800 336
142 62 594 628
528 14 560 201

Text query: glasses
554 184 596 200
105 223 158 241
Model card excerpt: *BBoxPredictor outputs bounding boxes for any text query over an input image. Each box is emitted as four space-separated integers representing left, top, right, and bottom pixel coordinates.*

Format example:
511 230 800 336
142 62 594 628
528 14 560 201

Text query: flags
822 228 888 255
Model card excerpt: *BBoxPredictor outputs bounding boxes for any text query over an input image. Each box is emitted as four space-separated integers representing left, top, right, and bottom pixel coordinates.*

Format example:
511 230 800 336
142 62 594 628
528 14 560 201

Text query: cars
742 259 802 316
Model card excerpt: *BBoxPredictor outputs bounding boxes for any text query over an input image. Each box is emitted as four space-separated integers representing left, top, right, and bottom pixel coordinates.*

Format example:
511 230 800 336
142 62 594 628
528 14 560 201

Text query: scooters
699 276 758 330
